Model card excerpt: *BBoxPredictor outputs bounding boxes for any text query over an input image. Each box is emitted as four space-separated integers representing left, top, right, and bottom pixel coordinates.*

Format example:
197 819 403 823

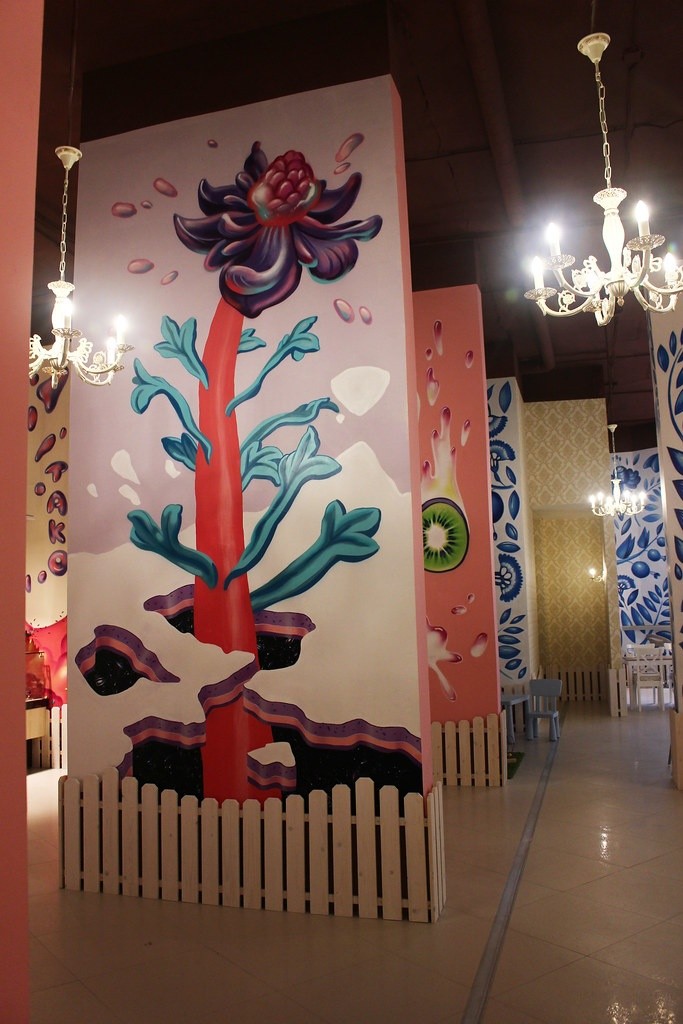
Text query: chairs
627 644 663 712
526 679 562 741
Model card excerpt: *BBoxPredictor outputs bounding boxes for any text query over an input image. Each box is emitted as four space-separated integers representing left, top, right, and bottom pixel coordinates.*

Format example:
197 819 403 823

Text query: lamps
523 0 683 326
30 0 135 389
591 326 647 516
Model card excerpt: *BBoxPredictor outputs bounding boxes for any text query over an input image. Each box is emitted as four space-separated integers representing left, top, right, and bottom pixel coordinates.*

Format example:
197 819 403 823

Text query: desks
501 695 531 743
622 656 673 711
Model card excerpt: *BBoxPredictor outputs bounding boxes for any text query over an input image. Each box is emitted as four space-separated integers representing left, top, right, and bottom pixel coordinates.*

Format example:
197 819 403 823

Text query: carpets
508 752 525 779
